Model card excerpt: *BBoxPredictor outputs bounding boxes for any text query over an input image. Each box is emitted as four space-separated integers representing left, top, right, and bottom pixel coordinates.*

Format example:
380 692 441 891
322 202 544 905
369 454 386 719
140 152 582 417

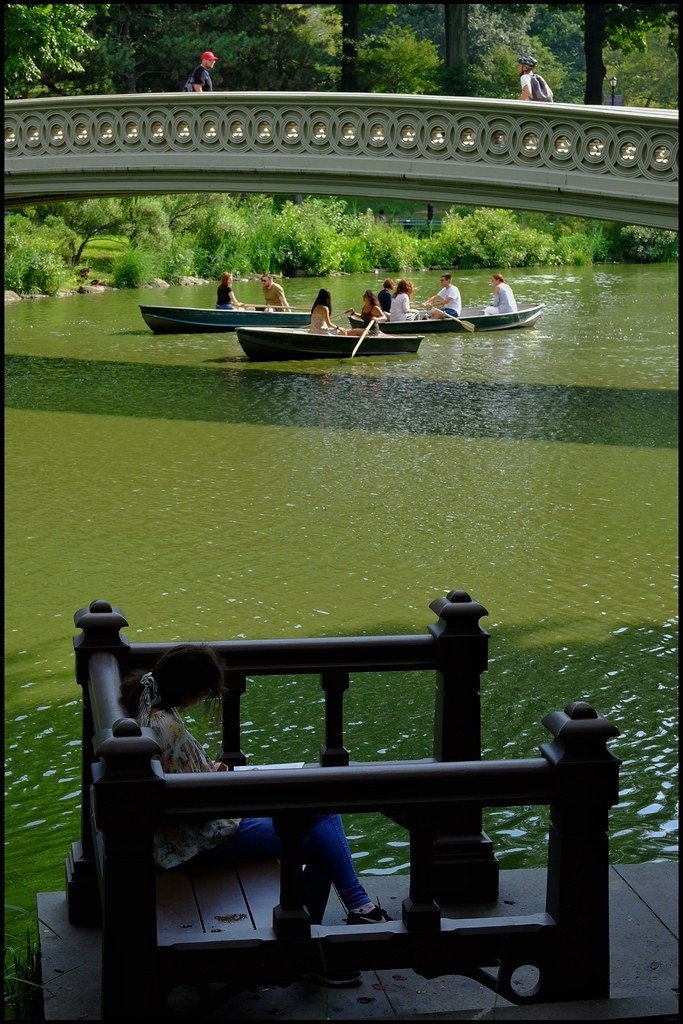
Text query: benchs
154 841 310 933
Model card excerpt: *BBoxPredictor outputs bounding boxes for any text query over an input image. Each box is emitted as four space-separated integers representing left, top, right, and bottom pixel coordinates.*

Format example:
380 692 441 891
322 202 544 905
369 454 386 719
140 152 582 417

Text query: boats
235 326 425 362
347 304 545 333
138 304 312 334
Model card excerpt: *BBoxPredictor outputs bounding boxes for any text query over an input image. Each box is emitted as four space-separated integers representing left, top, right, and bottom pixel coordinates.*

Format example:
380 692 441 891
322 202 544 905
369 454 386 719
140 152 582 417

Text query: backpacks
183 78 194 92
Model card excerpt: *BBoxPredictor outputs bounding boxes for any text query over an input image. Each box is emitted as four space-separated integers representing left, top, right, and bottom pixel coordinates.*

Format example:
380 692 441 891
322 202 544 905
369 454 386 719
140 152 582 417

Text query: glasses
440 279 444 281
262 280 266 282
362 295 366 297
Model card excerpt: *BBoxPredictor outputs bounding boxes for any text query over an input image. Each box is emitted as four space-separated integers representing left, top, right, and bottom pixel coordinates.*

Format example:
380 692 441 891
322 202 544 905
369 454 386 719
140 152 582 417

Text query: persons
311 273 461 336
216 272 244 310
191 52 218 92
19 90 31 99
427 202 434 229
260 273 292 312
484 273 518 315
120 644 393 985
379 210 387 226
515 56 538 101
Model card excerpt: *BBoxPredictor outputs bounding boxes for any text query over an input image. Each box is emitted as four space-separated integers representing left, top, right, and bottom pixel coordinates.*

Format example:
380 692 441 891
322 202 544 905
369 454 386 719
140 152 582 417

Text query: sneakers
346 896 394 925
302 969 364 985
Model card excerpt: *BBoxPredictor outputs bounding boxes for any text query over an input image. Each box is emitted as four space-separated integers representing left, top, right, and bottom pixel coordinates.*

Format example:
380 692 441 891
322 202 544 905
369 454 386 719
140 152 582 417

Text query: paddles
350 320 374 359
431 306 475 332
243 303 312 313
301 308 354 328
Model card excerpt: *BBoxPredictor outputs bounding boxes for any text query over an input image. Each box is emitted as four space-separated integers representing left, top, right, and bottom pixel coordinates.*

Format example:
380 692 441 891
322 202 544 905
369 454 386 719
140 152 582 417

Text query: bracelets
336 326 339 330
351 312 355 315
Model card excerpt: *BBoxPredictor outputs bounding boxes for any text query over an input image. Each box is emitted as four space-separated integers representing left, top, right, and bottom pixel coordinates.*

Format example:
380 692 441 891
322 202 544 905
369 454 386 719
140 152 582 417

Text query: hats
201 51 219 60
